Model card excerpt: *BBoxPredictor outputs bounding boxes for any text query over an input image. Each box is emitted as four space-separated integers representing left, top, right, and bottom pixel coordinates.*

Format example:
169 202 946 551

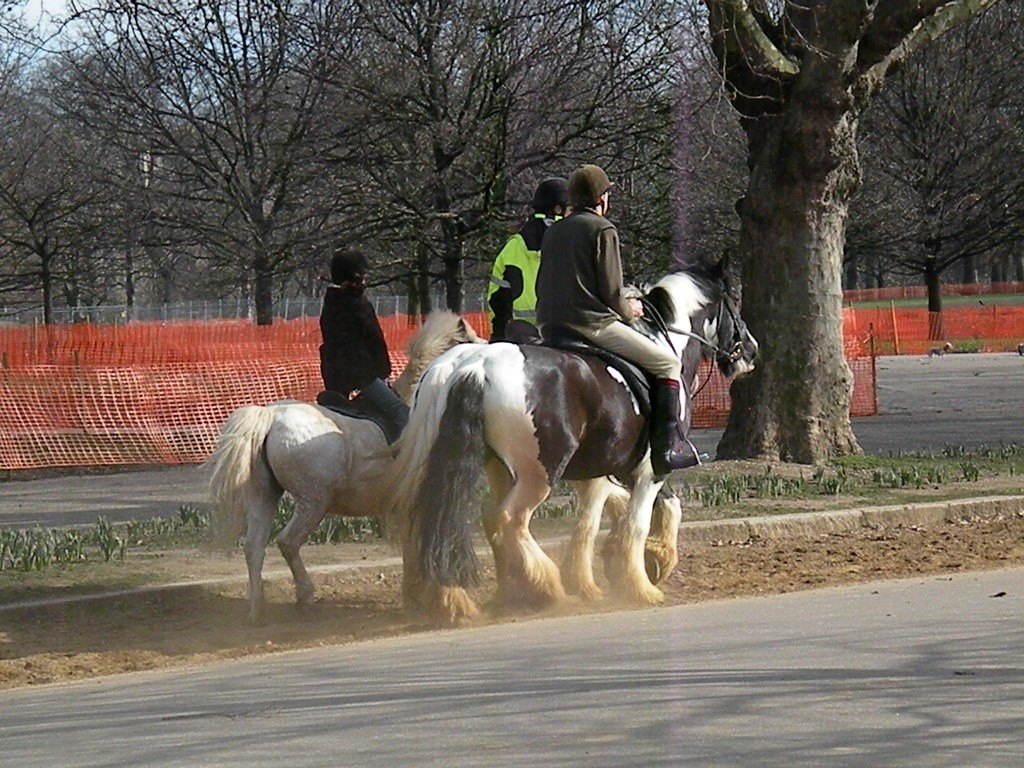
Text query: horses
197 249 759 627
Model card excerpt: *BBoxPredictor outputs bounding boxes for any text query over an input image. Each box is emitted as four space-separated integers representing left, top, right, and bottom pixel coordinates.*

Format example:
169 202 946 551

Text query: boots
649 387 709 476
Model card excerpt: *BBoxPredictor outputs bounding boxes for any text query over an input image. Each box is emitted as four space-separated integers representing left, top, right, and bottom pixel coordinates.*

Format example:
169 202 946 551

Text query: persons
319 251 410 433
488 177 573 344
535 165 708 474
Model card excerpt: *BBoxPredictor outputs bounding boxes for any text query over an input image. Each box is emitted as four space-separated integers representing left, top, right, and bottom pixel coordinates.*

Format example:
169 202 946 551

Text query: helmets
330 251 377 286
531 177 570 219
568 164 615 208
